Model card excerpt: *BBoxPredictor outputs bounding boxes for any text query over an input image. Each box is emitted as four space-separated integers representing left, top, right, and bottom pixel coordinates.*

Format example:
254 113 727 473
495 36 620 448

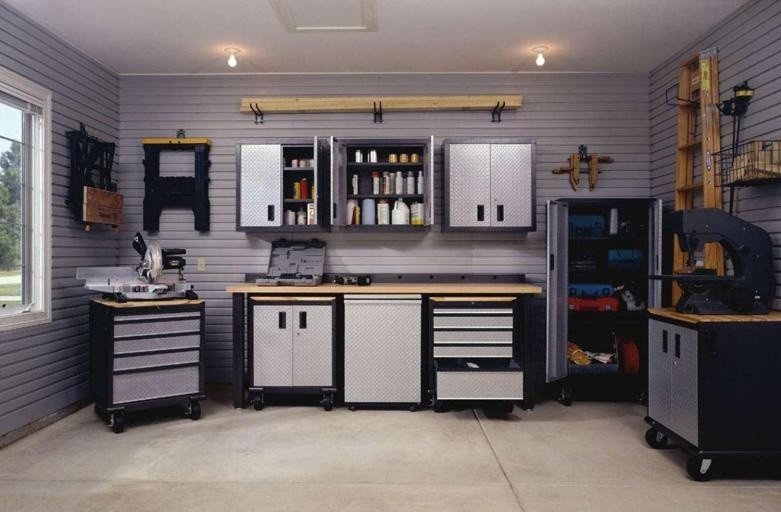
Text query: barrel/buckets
391 198 410 225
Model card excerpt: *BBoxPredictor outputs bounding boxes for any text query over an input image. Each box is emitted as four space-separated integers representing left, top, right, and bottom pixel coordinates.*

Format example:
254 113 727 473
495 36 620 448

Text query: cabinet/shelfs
231 133 544 419
85 136 214 436
544 133 781 482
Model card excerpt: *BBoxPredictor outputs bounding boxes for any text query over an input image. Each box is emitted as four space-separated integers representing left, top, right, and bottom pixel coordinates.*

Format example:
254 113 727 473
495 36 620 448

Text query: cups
388 154 420 163
292 159 313 168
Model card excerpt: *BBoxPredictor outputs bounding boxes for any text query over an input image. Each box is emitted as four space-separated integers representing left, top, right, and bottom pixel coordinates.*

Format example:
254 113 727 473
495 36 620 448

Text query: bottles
356 147 378 162
292 176 314 200
285 202 307 225
371 170 424 194
362 199 375 225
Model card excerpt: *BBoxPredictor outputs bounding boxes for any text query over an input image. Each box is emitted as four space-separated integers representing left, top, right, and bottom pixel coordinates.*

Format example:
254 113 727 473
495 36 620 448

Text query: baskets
710 140 781 187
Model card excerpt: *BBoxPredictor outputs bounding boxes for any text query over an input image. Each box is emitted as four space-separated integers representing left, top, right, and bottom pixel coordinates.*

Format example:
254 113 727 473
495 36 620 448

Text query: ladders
671 46 725 308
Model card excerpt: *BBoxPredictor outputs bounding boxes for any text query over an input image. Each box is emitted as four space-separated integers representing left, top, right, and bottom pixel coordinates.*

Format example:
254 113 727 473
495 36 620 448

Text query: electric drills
333 274 371 285
648 207 777 315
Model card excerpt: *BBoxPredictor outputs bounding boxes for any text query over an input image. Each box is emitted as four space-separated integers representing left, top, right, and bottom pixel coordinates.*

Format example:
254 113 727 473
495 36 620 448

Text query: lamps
531 46 550 67
223 46 241 68
